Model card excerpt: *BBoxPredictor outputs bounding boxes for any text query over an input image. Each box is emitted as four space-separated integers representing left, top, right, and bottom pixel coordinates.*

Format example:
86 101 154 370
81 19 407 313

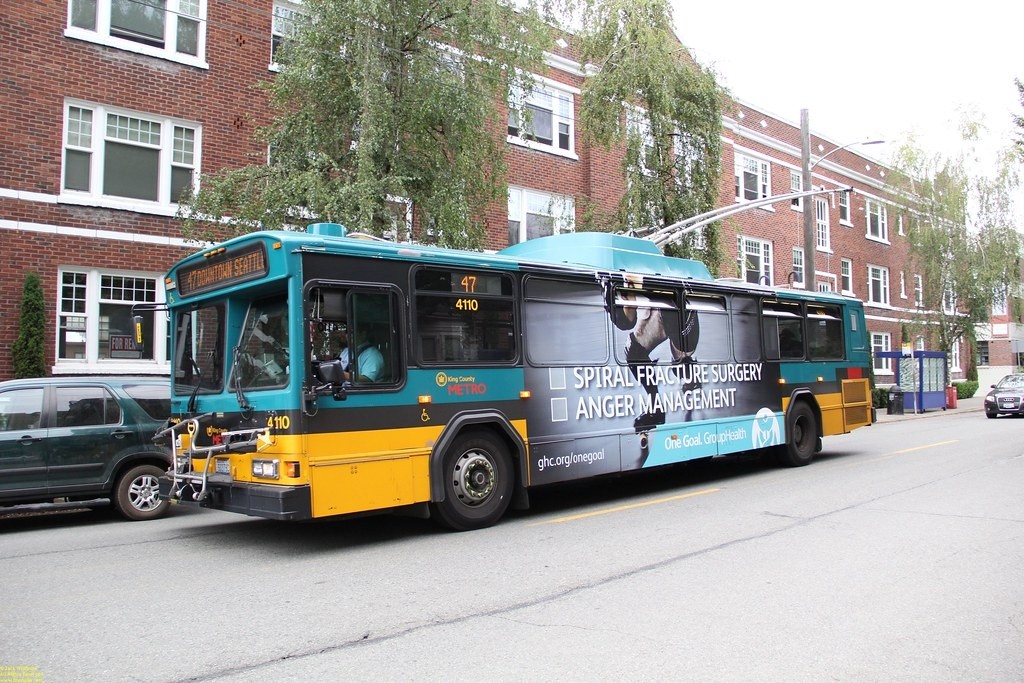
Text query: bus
130 187 877 531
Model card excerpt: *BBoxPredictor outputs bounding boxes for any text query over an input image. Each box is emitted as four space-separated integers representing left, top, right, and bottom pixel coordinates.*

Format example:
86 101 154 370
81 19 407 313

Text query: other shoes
634 406 665 427
682 378 701 394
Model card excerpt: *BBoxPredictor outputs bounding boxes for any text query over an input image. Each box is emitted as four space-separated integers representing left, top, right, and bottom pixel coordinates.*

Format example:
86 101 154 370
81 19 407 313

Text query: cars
984 374 1024 418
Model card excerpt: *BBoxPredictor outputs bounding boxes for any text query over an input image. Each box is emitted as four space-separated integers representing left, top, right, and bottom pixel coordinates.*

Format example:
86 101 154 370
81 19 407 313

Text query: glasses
345 329 362 334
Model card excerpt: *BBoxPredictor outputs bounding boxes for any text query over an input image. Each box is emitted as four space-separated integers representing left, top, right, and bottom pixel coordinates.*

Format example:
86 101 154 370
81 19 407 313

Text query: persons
336 319 387 385
253 340 276 382
594 271 701 435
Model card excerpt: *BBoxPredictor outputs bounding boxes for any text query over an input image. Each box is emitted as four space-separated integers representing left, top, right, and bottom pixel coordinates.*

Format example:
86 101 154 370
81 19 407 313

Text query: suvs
0 376 173 522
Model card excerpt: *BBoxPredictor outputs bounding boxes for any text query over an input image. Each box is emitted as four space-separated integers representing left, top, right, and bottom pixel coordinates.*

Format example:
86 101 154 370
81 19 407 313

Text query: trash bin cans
886 391 904 416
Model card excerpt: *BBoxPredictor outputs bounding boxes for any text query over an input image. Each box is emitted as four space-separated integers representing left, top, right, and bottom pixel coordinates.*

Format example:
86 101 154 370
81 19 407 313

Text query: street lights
800 108 885 291
